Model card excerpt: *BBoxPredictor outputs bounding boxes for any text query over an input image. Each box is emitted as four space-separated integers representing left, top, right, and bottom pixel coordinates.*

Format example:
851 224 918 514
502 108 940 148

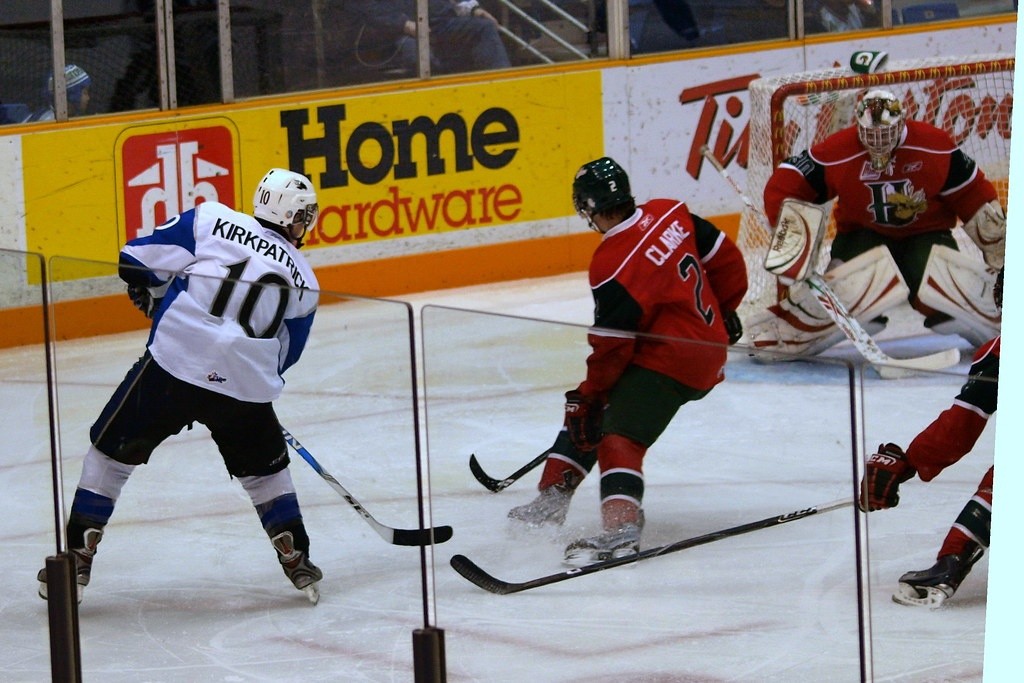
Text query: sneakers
892 544 984 609
507 469 584 527
270 526 323 606
37 528 104 604
564 508 645 566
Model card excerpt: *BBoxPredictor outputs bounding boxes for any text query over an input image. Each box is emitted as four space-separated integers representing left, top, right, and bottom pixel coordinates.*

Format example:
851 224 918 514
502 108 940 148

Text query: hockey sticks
449 494 863 598
127 284 455 548
696 143 962 382
469 445 556 494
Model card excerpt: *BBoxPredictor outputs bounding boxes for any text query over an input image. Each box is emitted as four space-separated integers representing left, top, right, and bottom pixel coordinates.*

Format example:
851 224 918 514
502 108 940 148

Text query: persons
128 0 544 109
22 65 91 123
596 0 882 54
508 157 749 557
745 90 1007 363
858 267 1004 599
37 168 323 589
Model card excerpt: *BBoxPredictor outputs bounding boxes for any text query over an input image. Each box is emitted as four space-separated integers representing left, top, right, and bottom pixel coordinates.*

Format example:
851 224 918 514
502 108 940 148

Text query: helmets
253 169 319 230
571 158 634 216
855 92 907 170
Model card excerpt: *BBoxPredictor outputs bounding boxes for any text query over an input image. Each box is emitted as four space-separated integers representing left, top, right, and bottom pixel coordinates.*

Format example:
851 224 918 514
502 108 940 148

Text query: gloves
562 388 606 454
859 442 916 513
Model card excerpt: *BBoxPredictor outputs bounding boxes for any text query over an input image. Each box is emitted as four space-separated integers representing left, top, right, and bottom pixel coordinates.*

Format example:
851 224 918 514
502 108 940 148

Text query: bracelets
471 6 481 16
